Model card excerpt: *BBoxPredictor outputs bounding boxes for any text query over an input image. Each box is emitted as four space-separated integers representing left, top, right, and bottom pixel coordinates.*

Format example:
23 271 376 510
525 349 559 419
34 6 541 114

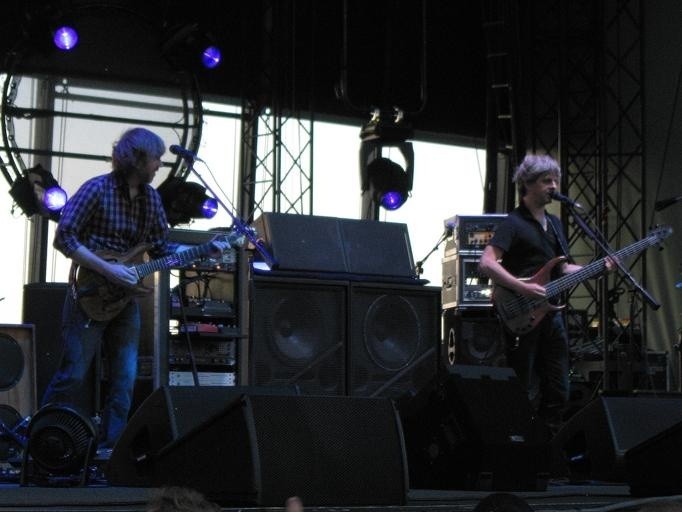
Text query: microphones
551 191 582 209
655 194 682 211
169 145 196 161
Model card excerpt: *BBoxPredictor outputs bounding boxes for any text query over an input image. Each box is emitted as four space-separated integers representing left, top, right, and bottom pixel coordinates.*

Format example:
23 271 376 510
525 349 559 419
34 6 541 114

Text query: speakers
24 282 68 410
547 398 682 482
144 395 410 506
627 422 682 495
348 281 442 397
249 276 349 396
0 325 38 463
249 212 416 278
108 385 300 487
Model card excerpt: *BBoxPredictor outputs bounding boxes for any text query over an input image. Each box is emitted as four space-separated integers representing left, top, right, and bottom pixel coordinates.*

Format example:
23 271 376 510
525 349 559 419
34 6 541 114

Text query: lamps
174 34 221 71
161 181 219 228
367 159 408 211
9 163 67 223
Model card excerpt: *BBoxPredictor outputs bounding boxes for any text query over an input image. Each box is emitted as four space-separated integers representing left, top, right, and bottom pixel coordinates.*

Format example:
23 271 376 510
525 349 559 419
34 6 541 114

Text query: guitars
69 226 258 321
492 222 673 335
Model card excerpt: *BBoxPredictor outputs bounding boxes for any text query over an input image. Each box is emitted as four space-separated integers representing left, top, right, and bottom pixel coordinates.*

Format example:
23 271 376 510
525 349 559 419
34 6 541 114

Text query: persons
475 149 625 492
29 128 233 487
145 486 306 512
